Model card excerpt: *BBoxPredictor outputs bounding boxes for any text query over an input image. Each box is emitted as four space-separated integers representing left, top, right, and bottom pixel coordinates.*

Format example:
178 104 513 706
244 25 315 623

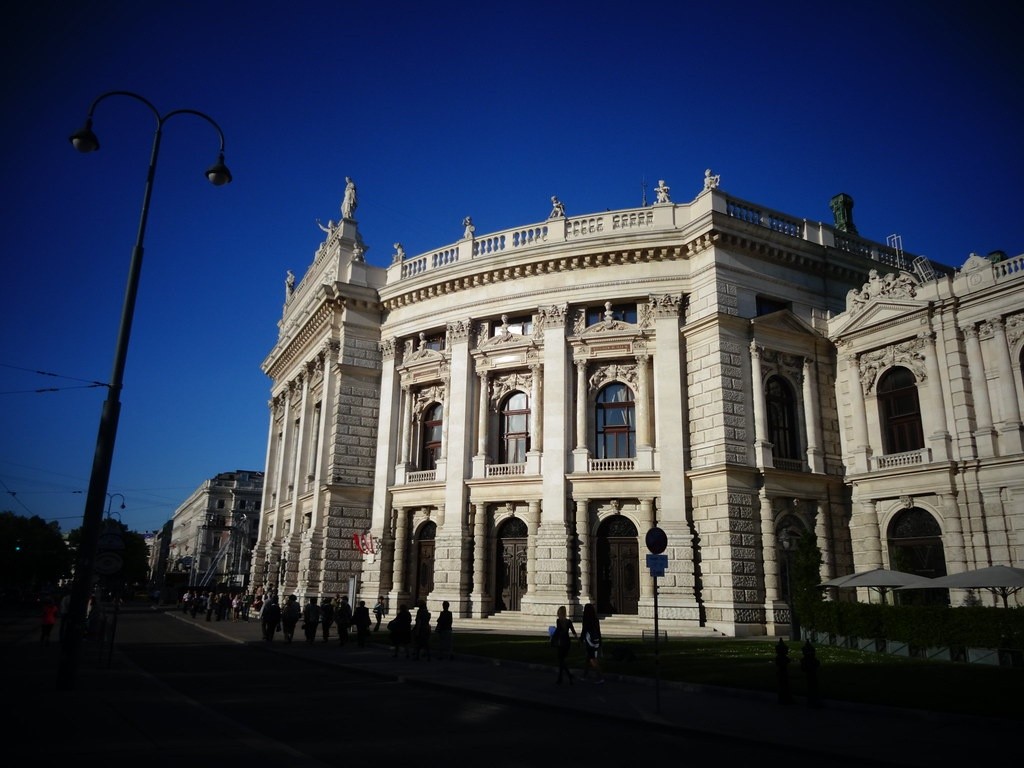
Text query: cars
88 593 121 614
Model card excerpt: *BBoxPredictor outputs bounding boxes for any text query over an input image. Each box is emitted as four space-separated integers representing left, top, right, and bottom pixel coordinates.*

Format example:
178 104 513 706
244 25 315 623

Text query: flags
352 532 368 555
361 532 377 555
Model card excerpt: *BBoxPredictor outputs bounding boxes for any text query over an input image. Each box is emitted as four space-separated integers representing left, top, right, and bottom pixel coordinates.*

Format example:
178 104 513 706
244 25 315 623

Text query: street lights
60 90 233 644
105 493 125 520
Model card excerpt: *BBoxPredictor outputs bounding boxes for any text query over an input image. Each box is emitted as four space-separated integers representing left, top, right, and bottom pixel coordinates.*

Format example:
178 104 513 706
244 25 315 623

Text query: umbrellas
813 568 932 603
892 565 1024 608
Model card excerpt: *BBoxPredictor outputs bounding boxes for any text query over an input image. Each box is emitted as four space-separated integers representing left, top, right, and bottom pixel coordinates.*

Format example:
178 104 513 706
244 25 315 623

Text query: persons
260 595 352 645
548 196 564 219
177 587 278 623
704 169 717 190
393 243 406 263
341 177 357 218
413 600 431 663
654 180 670 203
462 216 475 238
352 243 364 259
317 219 337 241
437 601 454 661
577 604 605 685
391 604 412 659
373 596 385 631
39 599 58 644
285 268 294 301
549 606 578 684
353 601 371 646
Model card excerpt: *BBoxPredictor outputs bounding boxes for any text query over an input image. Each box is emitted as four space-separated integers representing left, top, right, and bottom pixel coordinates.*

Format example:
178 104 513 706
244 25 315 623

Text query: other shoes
555 679 563 685
412 658 420 661
593 680 605 684
406 655 410 658
392 654 398 657
